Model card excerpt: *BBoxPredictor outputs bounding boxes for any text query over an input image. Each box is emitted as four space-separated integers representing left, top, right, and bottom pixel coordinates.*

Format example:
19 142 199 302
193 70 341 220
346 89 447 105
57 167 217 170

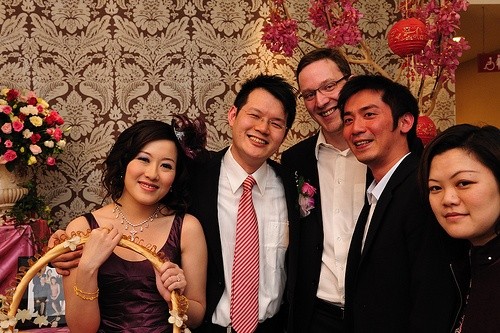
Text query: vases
0 164 29 226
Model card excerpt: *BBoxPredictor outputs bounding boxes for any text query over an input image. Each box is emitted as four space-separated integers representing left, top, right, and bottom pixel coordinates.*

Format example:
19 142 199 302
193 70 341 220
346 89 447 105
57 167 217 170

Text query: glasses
239 107 288 132
298 74 350 102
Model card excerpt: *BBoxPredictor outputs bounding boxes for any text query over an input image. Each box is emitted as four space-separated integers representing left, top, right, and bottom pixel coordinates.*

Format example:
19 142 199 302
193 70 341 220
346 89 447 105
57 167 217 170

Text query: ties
230 176 261 333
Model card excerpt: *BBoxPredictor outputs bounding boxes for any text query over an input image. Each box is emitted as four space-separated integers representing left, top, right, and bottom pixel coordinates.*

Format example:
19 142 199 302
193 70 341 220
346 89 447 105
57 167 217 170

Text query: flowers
260 0 470 117
0 87 67 168
299 177 317 218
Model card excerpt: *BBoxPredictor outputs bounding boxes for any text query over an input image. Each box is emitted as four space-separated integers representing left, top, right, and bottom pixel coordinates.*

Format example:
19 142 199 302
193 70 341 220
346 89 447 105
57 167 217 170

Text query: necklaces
112 202 161 237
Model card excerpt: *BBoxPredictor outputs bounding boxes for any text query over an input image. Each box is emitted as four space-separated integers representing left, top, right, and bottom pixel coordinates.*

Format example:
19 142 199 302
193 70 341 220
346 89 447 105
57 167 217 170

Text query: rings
175 275 180 282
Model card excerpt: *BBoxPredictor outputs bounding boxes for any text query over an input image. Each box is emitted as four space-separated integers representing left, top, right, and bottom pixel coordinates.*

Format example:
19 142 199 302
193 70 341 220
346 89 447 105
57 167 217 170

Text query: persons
31 270 65 314
337 76 468 333
65 121 208 333
415 124 500 333
282 49 424 333
45 75 314 333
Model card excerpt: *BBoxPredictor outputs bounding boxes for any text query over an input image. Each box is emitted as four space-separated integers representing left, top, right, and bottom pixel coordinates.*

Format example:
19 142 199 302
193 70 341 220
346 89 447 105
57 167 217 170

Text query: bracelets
72 285 99 301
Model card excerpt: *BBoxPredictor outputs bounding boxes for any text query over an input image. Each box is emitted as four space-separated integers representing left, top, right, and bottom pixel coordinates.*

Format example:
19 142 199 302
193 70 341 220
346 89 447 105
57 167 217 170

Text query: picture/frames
15 256 67 329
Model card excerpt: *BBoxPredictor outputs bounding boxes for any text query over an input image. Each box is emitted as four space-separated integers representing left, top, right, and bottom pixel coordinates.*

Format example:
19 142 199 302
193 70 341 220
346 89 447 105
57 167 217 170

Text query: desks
0 217 52 295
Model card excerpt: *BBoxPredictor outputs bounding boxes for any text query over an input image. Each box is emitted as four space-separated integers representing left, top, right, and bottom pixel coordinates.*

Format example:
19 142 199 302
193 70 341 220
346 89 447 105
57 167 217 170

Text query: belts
211 324 260 333
318 300 344 319
